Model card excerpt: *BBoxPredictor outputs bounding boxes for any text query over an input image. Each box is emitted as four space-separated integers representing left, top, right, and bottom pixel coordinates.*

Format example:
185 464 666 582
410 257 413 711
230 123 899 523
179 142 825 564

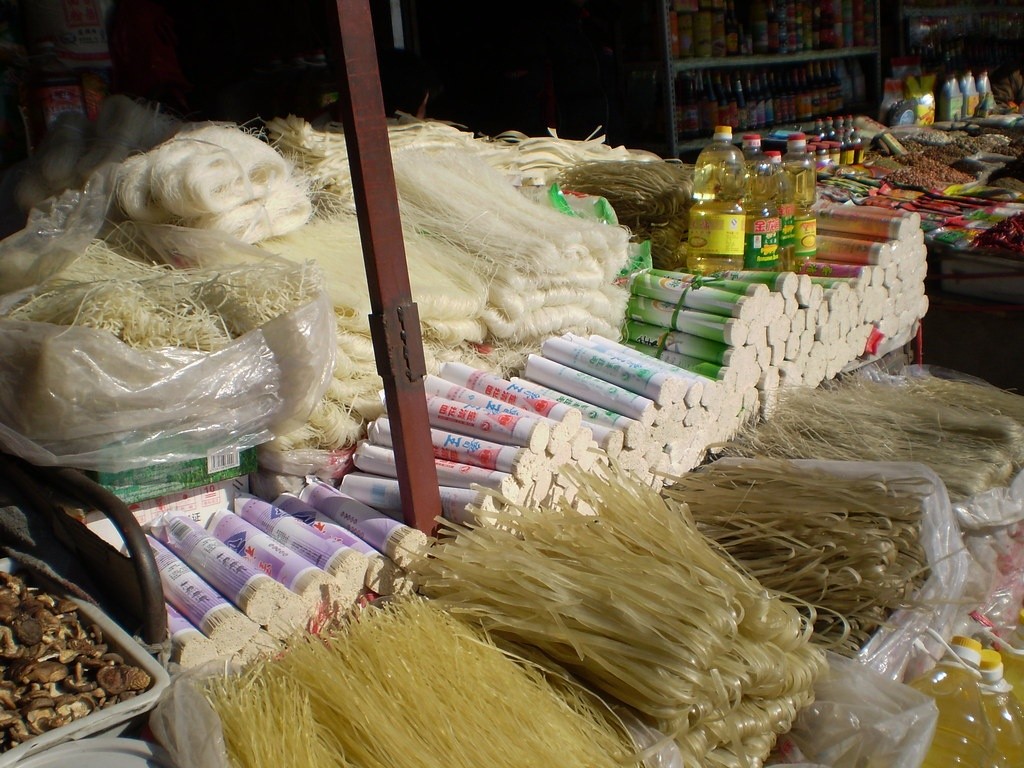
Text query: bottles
977 71 993 114
678 60 865 274
959 70 979 118
974 92 990 117
940 75 962 120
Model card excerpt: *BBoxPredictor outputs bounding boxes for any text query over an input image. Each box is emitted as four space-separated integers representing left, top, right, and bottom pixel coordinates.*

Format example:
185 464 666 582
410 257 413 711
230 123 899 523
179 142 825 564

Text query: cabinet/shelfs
616 1 881 163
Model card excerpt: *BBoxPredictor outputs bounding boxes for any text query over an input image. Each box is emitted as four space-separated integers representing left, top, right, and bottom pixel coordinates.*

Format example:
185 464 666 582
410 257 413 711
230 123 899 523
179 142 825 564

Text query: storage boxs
84 448 261 554
